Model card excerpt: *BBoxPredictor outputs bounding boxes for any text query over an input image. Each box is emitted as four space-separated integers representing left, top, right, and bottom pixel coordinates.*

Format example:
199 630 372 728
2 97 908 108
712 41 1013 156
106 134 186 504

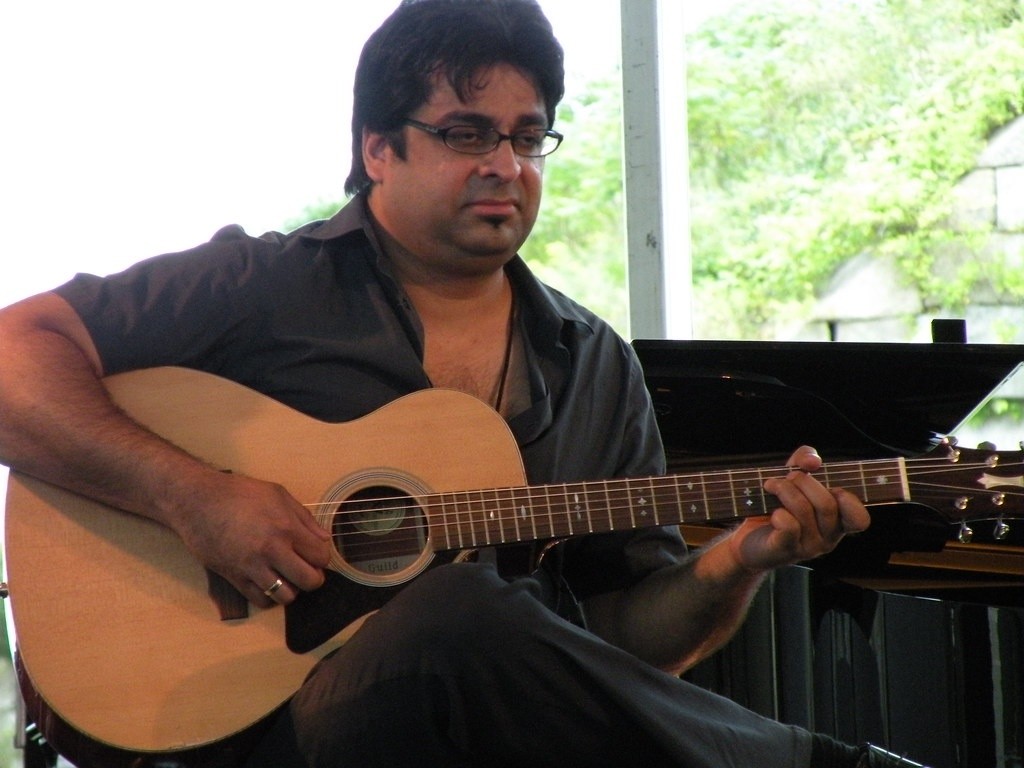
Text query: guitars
0 358 1024 768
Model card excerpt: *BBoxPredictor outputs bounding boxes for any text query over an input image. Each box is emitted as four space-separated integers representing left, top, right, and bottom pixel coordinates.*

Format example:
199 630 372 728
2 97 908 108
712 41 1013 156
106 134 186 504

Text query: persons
0 0 929 768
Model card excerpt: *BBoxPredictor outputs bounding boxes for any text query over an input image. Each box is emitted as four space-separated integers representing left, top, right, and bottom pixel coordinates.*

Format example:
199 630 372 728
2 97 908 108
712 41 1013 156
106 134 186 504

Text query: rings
264 578 284 597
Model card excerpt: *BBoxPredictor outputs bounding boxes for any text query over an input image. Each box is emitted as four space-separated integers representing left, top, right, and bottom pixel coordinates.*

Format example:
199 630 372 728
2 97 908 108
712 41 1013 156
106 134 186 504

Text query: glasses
398 116 565 158
